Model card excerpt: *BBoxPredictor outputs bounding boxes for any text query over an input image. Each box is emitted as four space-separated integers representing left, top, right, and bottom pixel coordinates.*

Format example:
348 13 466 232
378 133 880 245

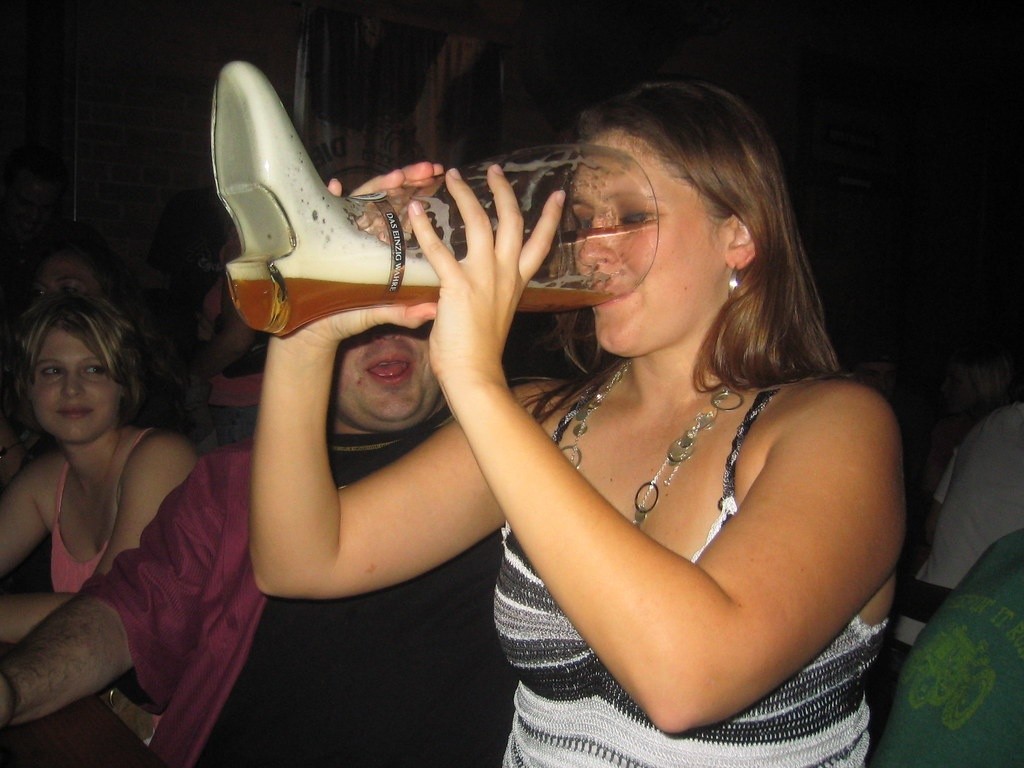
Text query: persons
0 143 269 500
849 327 1022 765
0 318 515 768
248 74 906 768
0 291 199 645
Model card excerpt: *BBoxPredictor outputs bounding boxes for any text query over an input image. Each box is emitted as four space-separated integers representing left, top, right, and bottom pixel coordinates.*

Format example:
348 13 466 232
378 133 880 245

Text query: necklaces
554 355 745 529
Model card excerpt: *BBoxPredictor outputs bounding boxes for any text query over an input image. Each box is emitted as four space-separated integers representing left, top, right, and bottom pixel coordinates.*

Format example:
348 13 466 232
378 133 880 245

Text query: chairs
863 573 954 758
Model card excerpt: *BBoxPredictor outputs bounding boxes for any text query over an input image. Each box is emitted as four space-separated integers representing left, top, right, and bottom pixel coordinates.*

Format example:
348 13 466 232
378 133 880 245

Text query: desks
0 572 173 768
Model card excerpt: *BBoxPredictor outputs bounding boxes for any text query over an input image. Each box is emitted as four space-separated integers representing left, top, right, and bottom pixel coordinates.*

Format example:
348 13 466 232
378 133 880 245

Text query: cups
210 60 658 337
